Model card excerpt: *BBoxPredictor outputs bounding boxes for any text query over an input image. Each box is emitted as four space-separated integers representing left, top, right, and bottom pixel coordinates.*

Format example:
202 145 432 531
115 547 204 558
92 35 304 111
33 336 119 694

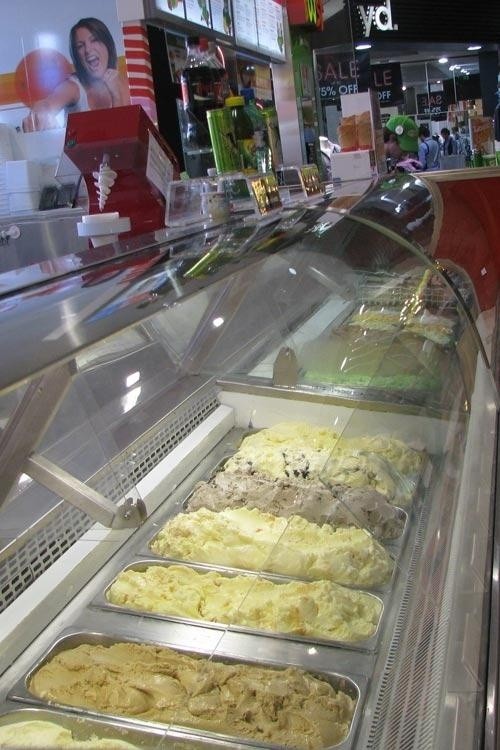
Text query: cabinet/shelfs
0 172 500 750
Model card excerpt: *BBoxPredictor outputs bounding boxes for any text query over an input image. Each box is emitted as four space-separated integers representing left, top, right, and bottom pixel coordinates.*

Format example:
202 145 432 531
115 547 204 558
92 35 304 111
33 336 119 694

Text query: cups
200 192 231 221
82 212 119 248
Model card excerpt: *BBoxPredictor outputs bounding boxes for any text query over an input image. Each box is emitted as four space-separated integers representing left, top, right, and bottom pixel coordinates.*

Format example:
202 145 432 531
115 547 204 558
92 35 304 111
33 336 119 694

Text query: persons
380 121 466 173
22 17 132 131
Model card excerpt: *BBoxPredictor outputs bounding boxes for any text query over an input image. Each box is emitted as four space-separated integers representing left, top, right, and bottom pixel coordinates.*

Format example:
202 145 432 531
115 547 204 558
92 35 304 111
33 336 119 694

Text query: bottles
181 36 272 173
464 155 470 168
